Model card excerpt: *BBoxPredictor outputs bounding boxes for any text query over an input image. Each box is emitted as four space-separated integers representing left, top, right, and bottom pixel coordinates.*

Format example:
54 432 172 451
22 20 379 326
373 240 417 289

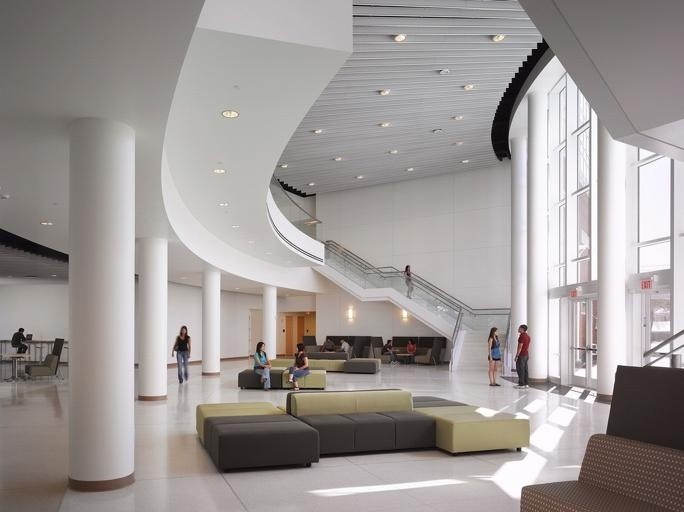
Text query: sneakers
514 385 531 389
261 376 300 392
489 382 501 387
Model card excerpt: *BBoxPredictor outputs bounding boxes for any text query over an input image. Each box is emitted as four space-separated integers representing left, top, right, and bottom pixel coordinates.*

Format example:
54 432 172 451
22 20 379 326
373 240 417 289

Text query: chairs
25 337 64 384
348 339 363 358
414 348 436 367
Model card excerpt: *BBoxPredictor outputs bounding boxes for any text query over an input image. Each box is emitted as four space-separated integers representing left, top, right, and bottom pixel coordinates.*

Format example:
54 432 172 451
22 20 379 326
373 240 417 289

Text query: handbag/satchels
174 342 179 352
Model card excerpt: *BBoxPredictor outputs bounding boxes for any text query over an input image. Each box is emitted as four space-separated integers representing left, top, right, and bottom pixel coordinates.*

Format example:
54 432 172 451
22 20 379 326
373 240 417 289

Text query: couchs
277 389 436 456
295 352 348 372
520 364 684 511
306 345 370 357
374 346 428 362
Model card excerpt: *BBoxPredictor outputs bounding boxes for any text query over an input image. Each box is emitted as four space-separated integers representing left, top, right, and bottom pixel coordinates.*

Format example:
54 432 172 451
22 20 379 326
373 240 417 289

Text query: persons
487 327 503 386
321 338 336 353
336 338 350 353
406 338 416 364
11 328 28 353
171 326 191 384
512 324 531 389
288 343 310 391
381 340 400 364
404 264 414 299
253 342 272 391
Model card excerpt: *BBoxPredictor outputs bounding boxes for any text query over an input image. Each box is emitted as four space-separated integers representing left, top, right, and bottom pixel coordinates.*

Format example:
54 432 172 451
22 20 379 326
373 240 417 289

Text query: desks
1 353 32 383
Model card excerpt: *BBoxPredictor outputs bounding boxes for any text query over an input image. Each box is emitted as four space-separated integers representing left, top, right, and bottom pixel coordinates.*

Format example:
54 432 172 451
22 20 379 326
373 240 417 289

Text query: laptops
26 334 32 340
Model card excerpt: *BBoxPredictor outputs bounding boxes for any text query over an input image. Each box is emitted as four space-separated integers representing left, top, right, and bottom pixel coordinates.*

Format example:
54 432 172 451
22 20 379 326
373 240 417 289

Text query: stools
238 368 327 390
271 359 295 368
413 397 530 455
195 401 320 472
344 358 381 374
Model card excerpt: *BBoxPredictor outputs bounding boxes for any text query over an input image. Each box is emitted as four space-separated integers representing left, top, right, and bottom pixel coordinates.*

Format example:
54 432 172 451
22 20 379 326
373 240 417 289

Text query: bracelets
516 355 518 357
